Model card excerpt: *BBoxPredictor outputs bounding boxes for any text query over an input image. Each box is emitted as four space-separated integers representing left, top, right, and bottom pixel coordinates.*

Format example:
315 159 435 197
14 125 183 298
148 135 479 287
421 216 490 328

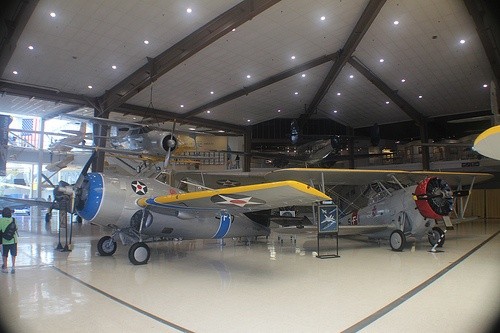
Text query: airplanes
264 165 494 252
0 145 333 265
48 108 215 164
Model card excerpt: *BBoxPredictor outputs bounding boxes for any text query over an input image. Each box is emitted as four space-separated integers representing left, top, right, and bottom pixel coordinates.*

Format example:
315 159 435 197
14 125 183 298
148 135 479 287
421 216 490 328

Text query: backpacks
3 221 16 240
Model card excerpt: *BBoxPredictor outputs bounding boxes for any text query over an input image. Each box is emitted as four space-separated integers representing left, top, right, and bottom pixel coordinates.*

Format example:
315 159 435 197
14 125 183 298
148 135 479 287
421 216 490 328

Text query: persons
0 207 19 273
227 154 240 170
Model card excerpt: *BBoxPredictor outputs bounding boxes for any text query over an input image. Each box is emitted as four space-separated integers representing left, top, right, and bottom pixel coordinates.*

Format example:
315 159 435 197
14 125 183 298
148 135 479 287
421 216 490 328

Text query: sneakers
2 268 9 272
11 268 16 273
